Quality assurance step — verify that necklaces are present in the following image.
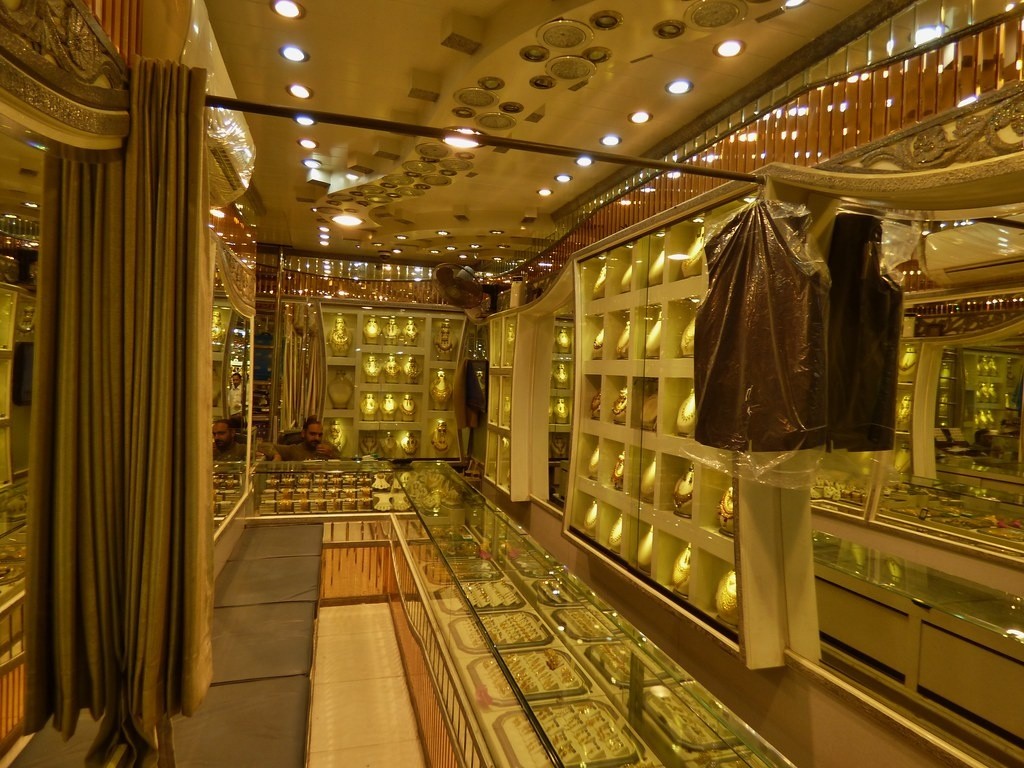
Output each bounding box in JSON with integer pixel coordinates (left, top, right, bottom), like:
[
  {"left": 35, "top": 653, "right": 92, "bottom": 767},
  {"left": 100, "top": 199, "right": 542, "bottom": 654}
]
[{"left": 331, "top": 237, "right": 737, "bottom": 612}]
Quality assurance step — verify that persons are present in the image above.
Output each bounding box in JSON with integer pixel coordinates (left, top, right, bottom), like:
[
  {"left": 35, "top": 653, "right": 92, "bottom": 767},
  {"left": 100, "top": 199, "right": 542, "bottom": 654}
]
[
  {"left": 258, "top": 415, "right": 342, "bottom": 461},
  {"left": 212, "top": 419, "right": 246, "bottom": 461},
  {"left": 228, "top": 373, "right": 248, "bottom": 421}
]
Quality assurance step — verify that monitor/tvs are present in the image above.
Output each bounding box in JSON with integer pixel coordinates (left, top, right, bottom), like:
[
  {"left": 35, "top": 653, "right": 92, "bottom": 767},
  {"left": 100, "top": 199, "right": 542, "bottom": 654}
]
[{"left": 467, "top": 359, "right": 489, "bottom": 413}]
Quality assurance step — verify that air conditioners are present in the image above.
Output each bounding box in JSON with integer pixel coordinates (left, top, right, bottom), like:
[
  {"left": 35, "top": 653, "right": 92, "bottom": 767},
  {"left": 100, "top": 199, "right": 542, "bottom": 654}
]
[{"left": 923, "top": 214, "right": 1024, "bottom": 288}]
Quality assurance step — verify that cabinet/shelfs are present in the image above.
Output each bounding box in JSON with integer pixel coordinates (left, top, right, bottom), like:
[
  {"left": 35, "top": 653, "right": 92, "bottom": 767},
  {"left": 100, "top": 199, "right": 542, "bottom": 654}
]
[
  {"left": 936, "top": 349, "right": 1024, "bottom": 432},
  {"left": 315, "top": 302, "right": 469, "bottom": 463},
  {"left": 490, "top": 173, "right": 785, "bottom": 671}
]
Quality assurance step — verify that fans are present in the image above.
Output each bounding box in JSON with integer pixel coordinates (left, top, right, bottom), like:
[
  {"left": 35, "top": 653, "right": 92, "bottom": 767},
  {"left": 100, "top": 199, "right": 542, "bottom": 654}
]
[{"left": 432, "top": 262, "right": 489, "bottom": 319}]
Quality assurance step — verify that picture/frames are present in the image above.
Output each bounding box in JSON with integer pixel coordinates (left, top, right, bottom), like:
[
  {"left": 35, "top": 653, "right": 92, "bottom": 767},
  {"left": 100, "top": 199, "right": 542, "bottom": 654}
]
[{"left": 466, "top": 360, "right": 488, "bottom": 412}]
